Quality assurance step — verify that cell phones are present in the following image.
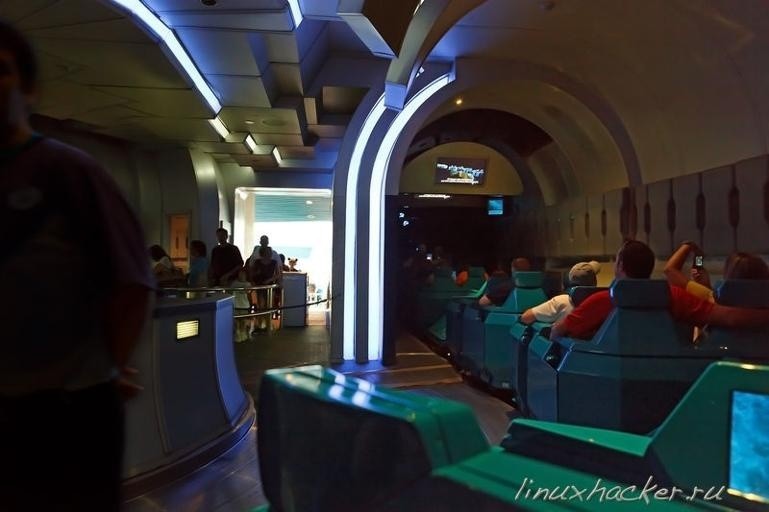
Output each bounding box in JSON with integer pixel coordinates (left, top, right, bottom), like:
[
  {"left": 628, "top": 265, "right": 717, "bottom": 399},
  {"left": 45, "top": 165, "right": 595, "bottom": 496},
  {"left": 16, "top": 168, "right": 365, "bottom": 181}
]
[{"left": 693, "top": 254, "right": 704, "bottom": 269}]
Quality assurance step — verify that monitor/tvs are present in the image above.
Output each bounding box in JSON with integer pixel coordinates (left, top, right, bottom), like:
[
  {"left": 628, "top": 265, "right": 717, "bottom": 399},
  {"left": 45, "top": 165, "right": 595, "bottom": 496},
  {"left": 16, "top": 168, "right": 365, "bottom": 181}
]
[
  {"left": 486, "top": 196, "right": 505, "bottom": 216},
  {"left": 724, "top": 387, "right": 769, "bottom": 504},
  {"left": 434, "top": 157, "right": 486, "bottom": 185}
]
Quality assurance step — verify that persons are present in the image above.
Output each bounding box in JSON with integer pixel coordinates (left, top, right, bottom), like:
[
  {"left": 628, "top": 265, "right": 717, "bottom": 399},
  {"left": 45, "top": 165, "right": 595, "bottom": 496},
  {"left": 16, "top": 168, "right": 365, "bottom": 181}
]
[
  {"left": 0, "top": 15, "right": 156, "bottom": 512},
  {"left": 149, "top": 224, "right": 292, "bottom": 343}
]
